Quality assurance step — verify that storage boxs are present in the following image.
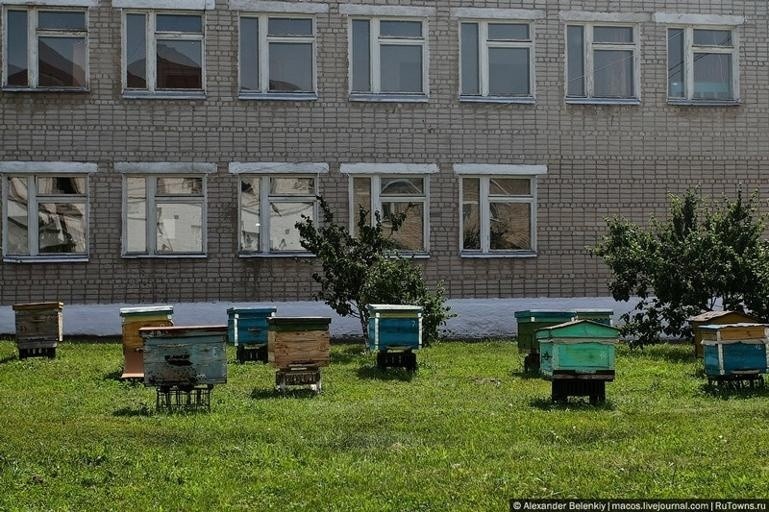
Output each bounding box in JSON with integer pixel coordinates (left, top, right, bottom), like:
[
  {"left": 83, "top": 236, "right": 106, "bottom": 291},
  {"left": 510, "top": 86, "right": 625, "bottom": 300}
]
[
  {"left": 119, "top": 304, "right": 175, "bottom": 345},
  {"left": 365, "top": 303, "right": 425, "bottom": 347},
  {"left": 227, "top": 305, "right": 277, "bottom": 344},
  {"left": 265, "top": 315, "right": 333, "bottom": 361},
  {"left": 138, "top": 323, "right": 230, "bottom": 385},
  {"left": 12, "top": 302, "right": 65, "bottom": 342},
  {"left": 516, "top": 309, "right": 614, "bottom": 352},
  {"left": 698, "top": 323, "right": 768, "bottom": 376},
  {"left": 538, "top": 319, "right": 621, "bottom": 380},
  {"left": 690, "top": 310, "right": 761, "bottom": 359}
]
